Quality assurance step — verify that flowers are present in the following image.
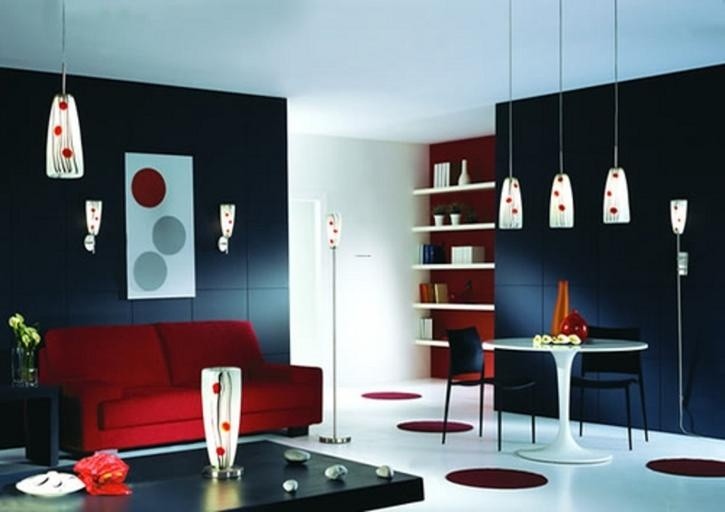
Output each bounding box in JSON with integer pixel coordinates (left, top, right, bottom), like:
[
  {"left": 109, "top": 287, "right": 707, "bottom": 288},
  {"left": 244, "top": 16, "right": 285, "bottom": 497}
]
[{"left": 8, "top": 313, "right": 42, "bottom": 379}]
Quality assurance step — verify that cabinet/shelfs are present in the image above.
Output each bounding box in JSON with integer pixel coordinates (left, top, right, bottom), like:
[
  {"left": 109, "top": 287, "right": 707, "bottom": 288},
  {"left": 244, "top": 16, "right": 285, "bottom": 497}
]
[{"left": 412, "top": 181, "right": 495, "bottom": 352}]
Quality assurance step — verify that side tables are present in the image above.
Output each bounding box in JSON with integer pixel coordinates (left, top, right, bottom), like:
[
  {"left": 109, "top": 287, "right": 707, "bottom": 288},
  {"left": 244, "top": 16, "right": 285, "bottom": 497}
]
[{"left": 0, "top": 383, "right": 60, "bottom": 467}]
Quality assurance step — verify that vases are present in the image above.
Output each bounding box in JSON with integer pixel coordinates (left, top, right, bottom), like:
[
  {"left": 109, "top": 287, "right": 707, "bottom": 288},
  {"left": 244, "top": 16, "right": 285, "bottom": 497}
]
[
  {"left": 458, "top": 160, "right": 471, "bottom": 185},
  {"left": 11, "top": 347, "right": 39, "bottom": 389},
  {"left": 562, "top": 308, "right": 589, "bottom": 343},
  {"left": 551, "top": 281, "right": 569, "bottom": 337}
]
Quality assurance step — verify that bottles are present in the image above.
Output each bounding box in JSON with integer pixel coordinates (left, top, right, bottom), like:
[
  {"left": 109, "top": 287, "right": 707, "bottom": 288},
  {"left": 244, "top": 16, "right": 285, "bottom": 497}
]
[
  {"left": 459, "top": 160, "right": 470, "bottom": 186},
  {"left": 554, "top": 281, "right": 569, "bottom": 335}
]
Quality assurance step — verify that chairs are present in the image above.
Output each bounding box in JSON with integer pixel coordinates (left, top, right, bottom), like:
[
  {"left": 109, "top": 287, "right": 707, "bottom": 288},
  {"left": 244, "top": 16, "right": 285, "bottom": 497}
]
[
  {"left": 572, "top": 327, "right": 648, "bottom": 450},
  {"left": 443, "top": 326, "right": 537, "bottom": 451}
]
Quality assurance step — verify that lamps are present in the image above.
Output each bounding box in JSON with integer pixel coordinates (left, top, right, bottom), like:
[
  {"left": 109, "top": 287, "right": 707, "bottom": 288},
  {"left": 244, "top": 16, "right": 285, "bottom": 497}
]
[
  {"left": 498, "top": 0, "right": 523, "bottom": 229},
  {"left": 217, "top": 204, "right": 236, "bottom": 252},
  {"left": 199, "top": 368, "right": 245, "bottom": 479},
  {"left": 83, "top": 200, "right": 103, "bottom": 255},
  {"left": 320, "top": 212, "right": 351, "bottom": 443},
  {"left": 671, "top": 199, "right": 688, "bottom": 433},
  {"left": 45, "top": 0, "right": 85, "bottom": 179},
  {"left": 548, "top": 0, "right": 574, "bottom": 229},
  {"left": 602, "top": 0, "right": 632, "bottom": 227}
]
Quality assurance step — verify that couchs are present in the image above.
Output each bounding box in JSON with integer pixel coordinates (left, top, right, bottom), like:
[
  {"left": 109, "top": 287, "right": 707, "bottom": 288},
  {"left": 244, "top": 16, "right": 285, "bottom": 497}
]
[{"left": 39, "top": 319, "right": 322, "bottom": 460}]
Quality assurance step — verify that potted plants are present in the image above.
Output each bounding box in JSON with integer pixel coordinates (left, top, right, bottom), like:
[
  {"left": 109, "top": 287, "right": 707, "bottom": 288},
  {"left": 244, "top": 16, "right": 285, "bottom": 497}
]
[
  {"left": 446, "top": 201, "right": 463, "bottom": 225},
  {"left": 432, "top": 206, "right": 445, "bottom": 226}
]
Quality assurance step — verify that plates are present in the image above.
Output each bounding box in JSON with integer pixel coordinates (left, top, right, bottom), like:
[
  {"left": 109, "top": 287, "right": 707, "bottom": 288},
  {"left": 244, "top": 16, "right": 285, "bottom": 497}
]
[{"left": 15, "top": 472, "right": 84, "bottom": 499}]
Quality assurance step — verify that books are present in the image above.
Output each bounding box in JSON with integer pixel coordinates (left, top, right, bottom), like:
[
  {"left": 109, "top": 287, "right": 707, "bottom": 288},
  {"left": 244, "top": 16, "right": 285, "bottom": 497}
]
[
  {"left": 451, "top": 245, "right": 486, "bottom": 265},
  {"left": 420, "top": 283, "right": 449, "bottom": 303},
  {"left": 419, "top": 244, "right": 446, "bottom": 264},
  {"left": 420, "top": 318, "right": 442, "bottom": 340},
  {"left": 433, "top": 162, "right": 460, "bottom": 189}
]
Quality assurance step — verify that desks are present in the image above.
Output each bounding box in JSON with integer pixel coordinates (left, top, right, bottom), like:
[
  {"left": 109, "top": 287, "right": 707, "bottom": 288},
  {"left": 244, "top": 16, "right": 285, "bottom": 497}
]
[
  {"left": 0, "top": 439, "right": 424, "bottom": 512},
  {"left": 481, "top": 338, "right": 649, "bottom": 464}
]
